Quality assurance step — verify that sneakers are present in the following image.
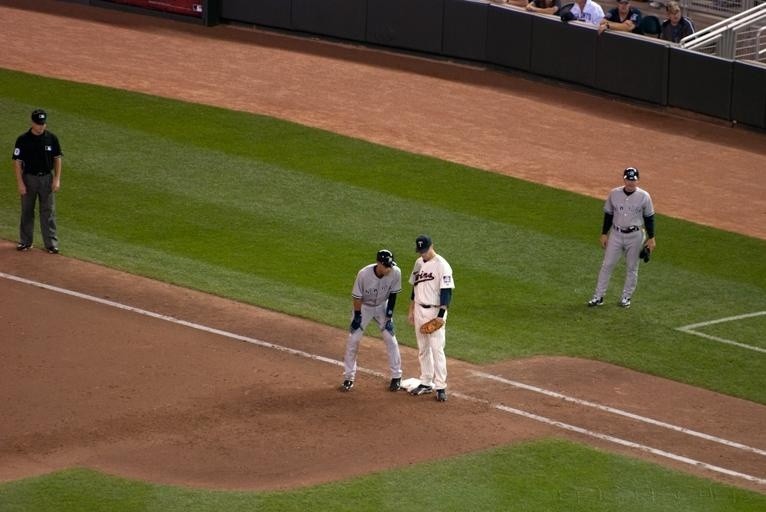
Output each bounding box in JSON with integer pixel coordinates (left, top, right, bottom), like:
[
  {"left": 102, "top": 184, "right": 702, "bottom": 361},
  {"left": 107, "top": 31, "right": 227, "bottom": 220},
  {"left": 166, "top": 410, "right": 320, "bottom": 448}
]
[
  {"left": 390, "top": 378, "right": 401, "bottom": 392},
  {"left": 617, "top": 297, "right": 631, "bottom": 306},
  {"left": 45, "top": 244, "right": 59, "bottom": 254},
  {"left": 17, "top": 242, "right": 34, "bottom": 251},
  {"left": 409, "top": 384, "right": 433, "bottom": 396},
  {"left": 342, "top": 379, "right": 354, "bottom": 391},
  {"left": 587, "top": 296, "right": 605, "bottom": 306},
  {"left": 435, "top": 387, "right": 448, "bottom": 402}
]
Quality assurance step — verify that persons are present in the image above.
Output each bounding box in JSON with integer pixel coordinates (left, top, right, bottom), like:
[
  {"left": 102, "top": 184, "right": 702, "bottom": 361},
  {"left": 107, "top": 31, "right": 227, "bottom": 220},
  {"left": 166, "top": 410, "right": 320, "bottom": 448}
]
[
  {"left": 339, "top": 249, "right": 404, "bottom": 393},
  {"left": 588, "top": 166, "right": 656, "bottom": 310},
  {"left": 526, "top": 0, "right": 561, "bottom": 16},
  {"left": 597, "top": 0, "right": 644, "bottom": 38},
  {"left": 407, "top": 234, "right": 456, "bottom": 402},
  {"left": 661, "top": 0, "right": 697, "bottom": 44},
  {"left": 12, "top": 109, "right": 64, "bottom": 254},
  {"left": 570, "top": 0, "right": 605, "bottom": 26}
]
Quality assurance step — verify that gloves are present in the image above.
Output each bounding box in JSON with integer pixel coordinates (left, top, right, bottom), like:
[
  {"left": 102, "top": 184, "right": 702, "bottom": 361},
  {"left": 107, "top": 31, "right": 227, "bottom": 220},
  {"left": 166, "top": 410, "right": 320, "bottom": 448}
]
[
  {"left": 349, "top": 310, "right": 363, "bottom": 334},
  {"left": 384, "top": 317, "right": 396, "bottom": 336}
]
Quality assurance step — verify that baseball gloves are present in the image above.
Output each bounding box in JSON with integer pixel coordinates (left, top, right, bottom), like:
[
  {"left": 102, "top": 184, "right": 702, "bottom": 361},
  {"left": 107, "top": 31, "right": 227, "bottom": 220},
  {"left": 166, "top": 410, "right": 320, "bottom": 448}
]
[
  {"left": 420, "top": 318, "right": 443, "bottom": 334},
  {"left": 638, "top": 243, "right": 653, "bottom": 262}
]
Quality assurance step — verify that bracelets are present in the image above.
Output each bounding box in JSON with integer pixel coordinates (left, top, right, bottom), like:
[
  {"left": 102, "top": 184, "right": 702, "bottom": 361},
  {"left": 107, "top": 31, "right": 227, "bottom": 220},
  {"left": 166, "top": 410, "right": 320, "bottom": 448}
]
[{"left": 437, "top": 308, "right": 445, "bottom": 318}]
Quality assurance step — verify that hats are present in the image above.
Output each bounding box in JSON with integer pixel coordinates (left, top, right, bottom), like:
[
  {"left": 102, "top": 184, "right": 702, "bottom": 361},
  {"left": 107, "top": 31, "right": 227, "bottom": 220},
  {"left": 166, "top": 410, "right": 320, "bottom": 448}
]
[
  {"left": 623, "top": 166, "right": 639, "bottom": 181},
  {"left": 31, "top": 109, "right": 46, "bottom": 125},
  {"left": 376, "top": 250, "right": 396, "bottom": 268},
  {"left": 561, "top": 10, "right": 578, "bottom": 22},
  {"left": 416, "top": 233, "right": 432, "bottom": 253}
]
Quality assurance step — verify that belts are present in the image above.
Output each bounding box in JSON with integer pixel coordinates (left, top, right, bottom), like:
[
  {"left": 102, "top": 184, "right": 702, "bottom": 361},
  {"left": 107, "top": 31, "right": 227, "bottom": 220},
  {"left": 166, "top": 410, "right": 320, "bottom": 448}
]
[{"left": 613, "top": 225, "right": 642, "bottom": 232}]
